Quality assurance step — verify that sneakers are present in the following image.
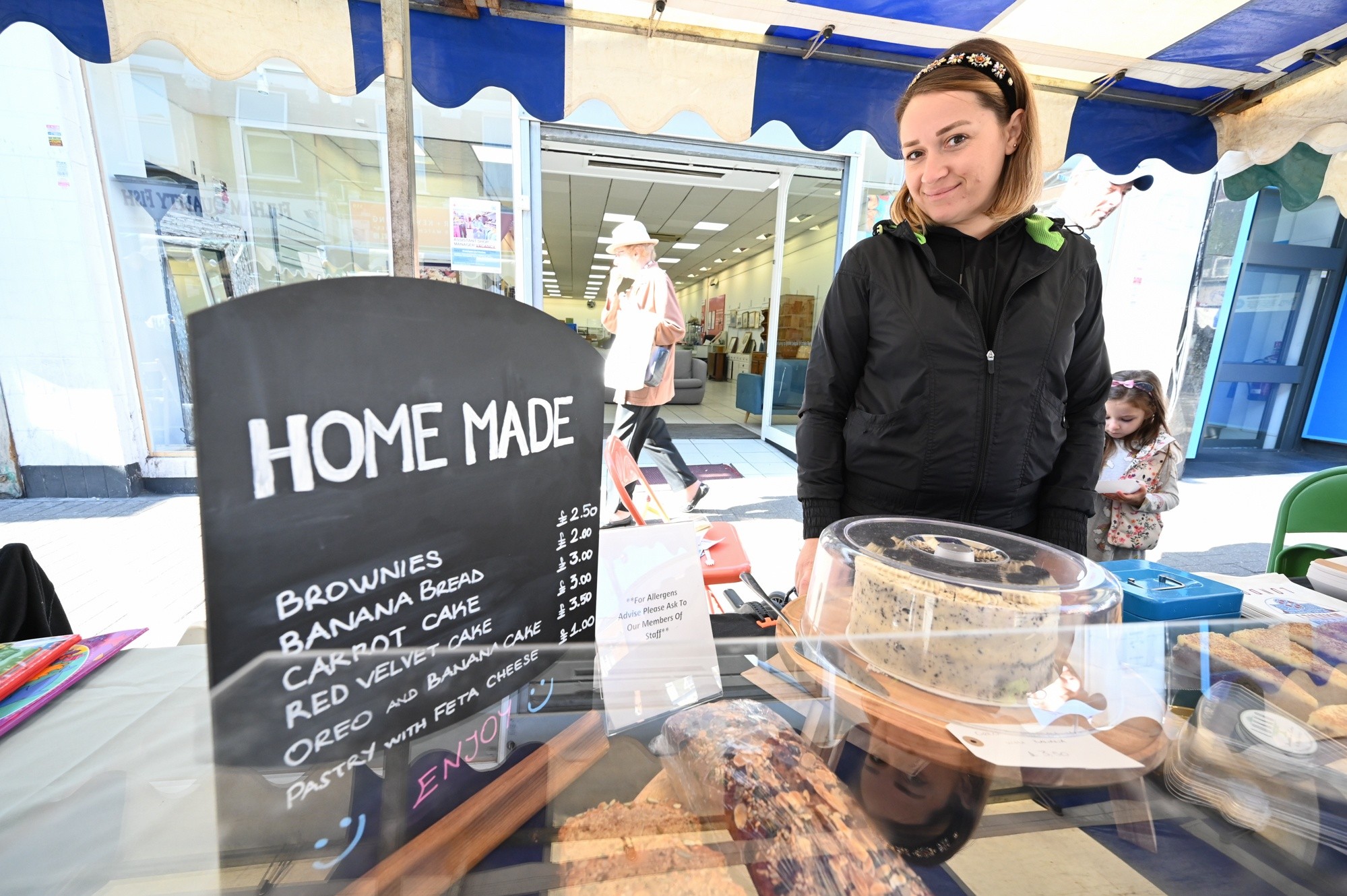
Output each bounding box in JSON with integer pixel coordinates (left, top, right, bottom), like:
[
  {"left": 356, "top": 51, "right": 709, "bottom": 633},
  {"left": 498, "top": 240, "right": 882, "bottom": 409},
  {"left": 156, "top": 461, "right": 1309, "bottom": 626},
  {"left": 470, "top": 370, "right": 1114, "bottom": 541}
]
[
  {"left": 598, "top": 513, "right": 634, "bottom": 529},
  {"left": 681, "top": 482, "right": 709, "bottom": 513}
]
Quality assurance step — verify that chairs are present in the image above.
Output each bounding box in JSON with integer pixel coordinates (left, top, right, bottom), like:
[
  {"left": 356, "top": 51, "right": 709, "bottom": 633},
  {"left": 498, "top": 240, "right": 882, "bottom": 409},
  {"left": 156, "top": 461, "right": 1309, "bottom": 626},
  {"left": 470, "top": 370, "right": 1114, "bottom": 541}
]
[
  {"left": 605, "top": 434, "right": 750, "bottom": 615},
  {"left": 1264, "top": 467, "right": 1347, "bottom": 578}
]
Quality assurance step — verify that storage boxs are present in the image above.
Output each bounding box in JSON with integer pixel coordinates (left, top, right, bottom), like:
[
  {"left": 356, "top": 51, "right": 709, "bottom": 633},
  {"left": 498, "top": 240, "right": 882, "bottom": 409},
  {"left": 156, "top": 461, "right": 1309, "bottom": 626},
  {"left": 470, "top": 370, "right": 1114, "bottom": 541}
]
[
  {"left": 1098, "top": 560, "right": 1245, "bottom": 627},
  {"left": 583, "top": 334, "right": 599, "bottom": 347}
]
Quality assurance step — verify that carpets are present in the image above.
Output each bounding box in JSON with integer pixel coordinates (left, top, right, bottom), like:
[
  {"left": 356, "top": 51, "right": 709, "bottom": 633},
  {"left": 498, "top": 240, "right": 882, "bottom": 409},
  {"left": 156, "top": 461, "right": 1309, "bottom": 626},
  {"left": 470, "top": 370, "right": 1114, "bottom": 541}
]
[
  {"left": 633, "top": 462, "right": 745, "bottom": 485},
  {"left": 603, "top": 424, "right": 761, "bottom": 439}
]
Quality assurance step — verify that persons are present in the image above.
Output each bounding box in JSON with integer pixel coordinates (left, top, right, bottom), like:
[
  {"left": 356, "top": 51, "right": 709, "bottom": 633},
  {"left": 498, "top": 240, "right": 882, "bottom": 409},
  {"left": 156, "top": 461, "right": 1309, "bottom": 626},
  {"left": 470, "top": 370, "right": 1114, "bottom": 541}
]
[
  {"left": 835, "top": 711, "right": 985, "bottom": 868},
  {"left": 794, "top": 39, "right": 1113, "bottom": 600},
  {"left": 1087, "top": 370, "right": 1184, "bottom": 562},
  {"left": 600, "top": 222, "right": 710, "bottom": 530},
  {"left": 1040, "top": 156, "right": 1155, "bottom": 240},
  {"left": 501, "top": 220, "right": 515, "bottom": 298},
  {"left": 454, "top": 211, "right": 486, "bottom": 239}
]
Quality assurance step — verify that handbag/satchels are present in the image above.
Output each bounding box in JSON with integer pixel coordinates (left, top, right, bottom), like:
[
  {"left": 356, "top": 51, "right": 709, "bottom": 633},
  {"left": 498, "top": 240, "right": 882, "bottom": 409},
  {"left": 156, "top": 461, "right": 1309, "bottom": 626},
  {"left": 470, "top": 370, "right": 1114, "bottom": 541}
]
[{"left": 645, "top": 343, "right": 675, "bottom": 388}]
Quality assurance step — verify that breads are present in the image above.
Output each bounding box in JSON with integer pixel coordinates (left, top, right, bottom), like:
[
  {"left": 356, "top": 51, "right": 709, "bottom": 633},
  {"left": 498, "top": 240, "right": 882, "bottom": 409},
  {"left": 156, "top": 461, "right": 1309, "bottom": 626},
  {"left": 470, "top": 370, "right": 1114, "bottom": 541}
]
[
  {"left": 548, "top": 695, "right": 931, "bottom": 896},
  {"left": 1172, "top": 619, "right": 1347, "bottom": 740}
]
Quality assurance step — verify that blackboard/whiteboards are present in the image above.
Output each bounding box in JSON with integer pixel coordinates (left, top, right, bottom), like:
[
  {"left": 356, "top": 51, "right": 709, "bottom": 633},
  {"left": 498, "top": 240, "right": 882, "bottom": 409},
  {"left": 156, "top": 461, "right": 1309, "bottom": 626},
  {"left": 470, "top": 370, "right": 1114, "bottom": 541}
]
[{"left": 186, "top": 275, "right": 603, "bottom": 896}]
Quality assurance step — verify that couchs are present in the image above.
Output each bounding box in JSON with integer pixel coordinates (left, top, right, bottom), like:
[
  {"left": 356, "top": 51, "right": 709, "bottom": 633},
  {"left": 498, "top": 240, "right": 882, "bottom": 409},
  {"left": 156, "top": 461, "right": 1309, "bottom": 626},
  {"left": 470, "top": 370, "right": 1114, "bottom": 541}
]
[
  {"left": 736, "top": 359, "right": 811, "bottom": 423},
  {"left": 666, "top": 349, "right": 707, "bottom": 405}
]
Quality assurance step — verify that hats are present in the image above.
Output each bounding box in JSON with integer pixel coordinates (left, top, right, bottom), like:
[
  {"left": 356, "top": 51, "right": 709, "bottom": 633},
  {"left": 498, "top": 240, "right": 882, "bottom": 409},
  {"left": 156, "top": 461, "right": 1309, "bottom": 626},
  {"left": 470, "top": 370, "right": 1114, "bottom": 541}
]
[{"left": 606, "top": 220, "right": 659, "bottom": 254}]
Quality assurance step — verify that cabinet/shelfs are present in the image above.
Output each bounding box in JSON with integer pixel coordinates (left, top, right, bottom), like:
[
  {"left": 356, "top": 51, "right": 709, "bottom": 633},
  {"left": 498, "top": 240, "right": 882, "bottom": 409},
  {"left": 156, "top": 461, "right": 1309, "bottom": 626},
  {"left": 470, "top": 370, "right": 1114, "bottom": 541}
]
[
  {"left": 739, "top": 362, "right": 749, "bottom": 372},
  {"left": 589, "top": 327, "right": 605, "bottom": 341},
  {"left": 727, "top": 361, "right": 732, "bottom": 378},
  {"left": 751, "top": 295, "right": 814, "bottom": 374},
  {"left": 707, "top": 352, "right": 727, "bottom": 382},
  {"left": 732, "top": 361, "right": 739, "bottom": 378}
]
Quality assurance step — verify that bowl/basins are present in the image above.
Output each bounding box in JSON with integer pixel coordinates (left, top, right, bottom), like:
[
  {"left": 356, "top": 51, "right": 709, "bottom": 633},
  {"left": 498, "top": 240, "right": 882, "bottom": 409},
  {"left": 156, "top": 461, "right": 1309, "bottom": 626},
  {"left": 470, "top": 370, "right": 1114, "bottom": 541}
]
[{"left": 1095, "top": 480, "right": 1145, "bottom": 494}]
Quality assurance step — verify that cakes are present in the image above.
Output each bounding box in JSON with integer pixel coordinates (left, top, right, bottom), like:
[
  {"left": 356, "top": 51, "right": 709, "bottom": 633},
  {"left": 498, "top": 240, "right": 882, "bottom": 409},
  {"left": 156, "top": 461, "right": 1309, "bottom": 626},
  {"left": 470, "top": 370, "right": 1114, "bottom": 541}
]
[{"left": 846, "top": 534, "right": 1060, "bottom": 705}]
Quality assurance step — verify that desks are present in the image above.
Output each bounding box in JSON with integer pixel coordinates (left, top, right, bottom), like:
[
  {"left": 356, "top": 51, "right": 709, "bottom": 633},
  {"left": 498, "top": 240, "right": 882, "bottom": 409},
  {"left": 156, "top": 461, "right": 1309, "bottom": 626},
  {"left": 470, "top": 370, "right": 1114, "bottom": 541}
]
[{"left": 0, "top": 636, "right": 331, "bottom": 896}]
